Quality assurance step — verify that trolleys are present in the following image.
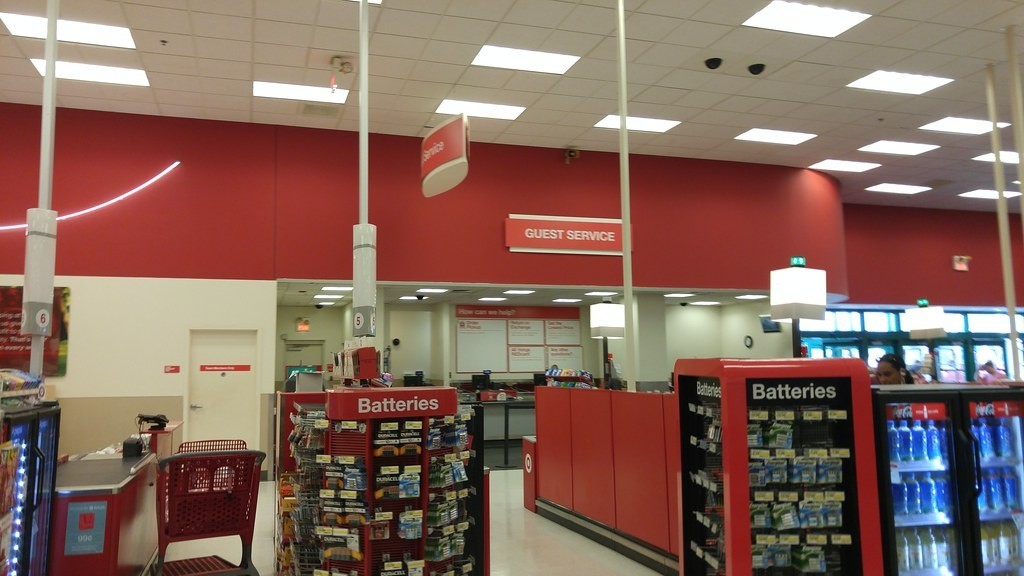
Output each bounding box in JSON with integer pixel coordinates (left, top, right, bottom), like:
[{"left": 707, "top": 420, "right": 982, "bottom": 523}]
[{"left": 150, "top": 439, "right": 267, "bottom": 576}]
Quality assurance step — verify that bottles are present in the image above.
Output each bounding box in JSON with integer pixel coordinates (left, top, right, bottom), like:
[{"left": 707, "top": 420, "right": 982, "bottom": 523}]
[
  {"left": 896, "top": 528, "right": 951, "bottom": 570},
  {"left": 888, "top": 419, "right": 948, "bottom": 461},
  {"left": 981, "top": 521, "right": 1019, "bottom": 566},
  {"left": 891, "top": 471, "right": 952, "bottom": 515},
  {"left": 971, "top": 417, "right": 1014, "bottom": 461},
  {"left": 975, "top": 467, "right": 1018, "bottom": 511}
]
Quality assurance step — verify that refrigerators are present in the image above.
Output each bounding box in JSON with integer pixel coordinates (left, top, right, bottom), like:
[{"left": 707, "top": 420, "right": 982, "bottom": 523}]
[{"left": 871, "top": 384, "right": 1024, "bottom": 576}]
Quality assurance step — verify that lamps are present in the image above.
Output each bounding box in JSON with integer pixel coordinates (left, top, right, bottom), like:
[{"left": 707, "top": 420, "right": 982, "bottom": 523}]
[
  {"left": 905, "top": 306, "right": 946, "bottom": 381},
  {"left": 769, "top": 266, "right": 827, "bottom": 358},
  {"left": 590, "top": 303, "right": 625, "bottom": 388}
]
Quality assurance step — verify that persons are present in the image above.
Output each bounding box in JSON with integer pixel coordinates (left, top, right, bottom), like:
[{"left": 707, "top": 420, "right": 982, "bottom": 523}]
[
  {"left": 877, "top": 352, "right": 915, "bottom": 384},
  {"left": 978, "top": 360, "right": 1008, "bottom": 383}
]
